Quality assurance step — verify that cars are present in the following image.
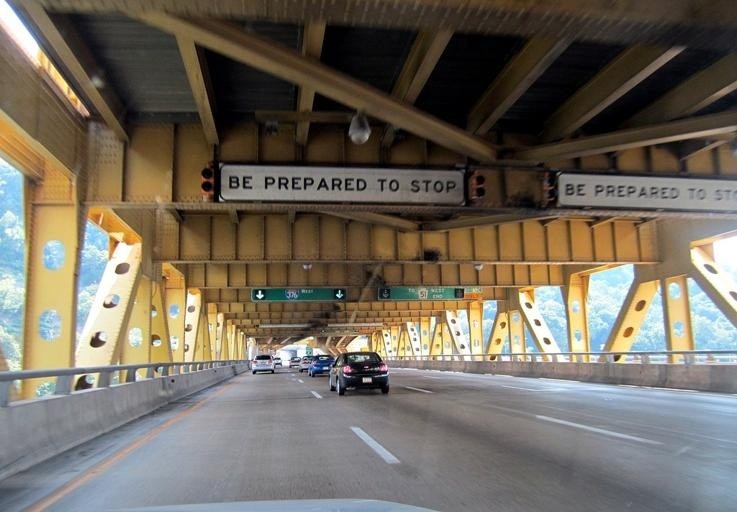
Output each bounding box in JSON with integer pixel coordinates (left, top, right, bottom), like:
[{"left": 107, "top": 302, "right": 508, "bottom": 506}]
[
  {"left": 251, "top": 354, "right": 335, "bottom": 377},
  {"left": 327, "top": 352, "right": 389, "bottom": 397}
]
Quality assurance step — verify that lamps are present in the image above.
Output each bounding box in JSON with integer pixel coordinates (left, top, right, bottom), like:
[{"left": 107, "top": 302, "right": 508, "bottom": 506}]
[{"left": 347, "top": 110, "right": 372, "bottom": 146}]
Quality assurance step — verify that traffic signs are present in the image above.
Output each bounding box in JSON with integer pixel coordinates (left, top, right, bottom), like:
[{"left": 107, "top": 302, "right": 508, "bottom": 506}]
[
  {"left": 376, "top": 286, "right": 483, "bottom": 301},
  {"left": 250, "top": 287, "right": 351, "bottom": 301}
]
